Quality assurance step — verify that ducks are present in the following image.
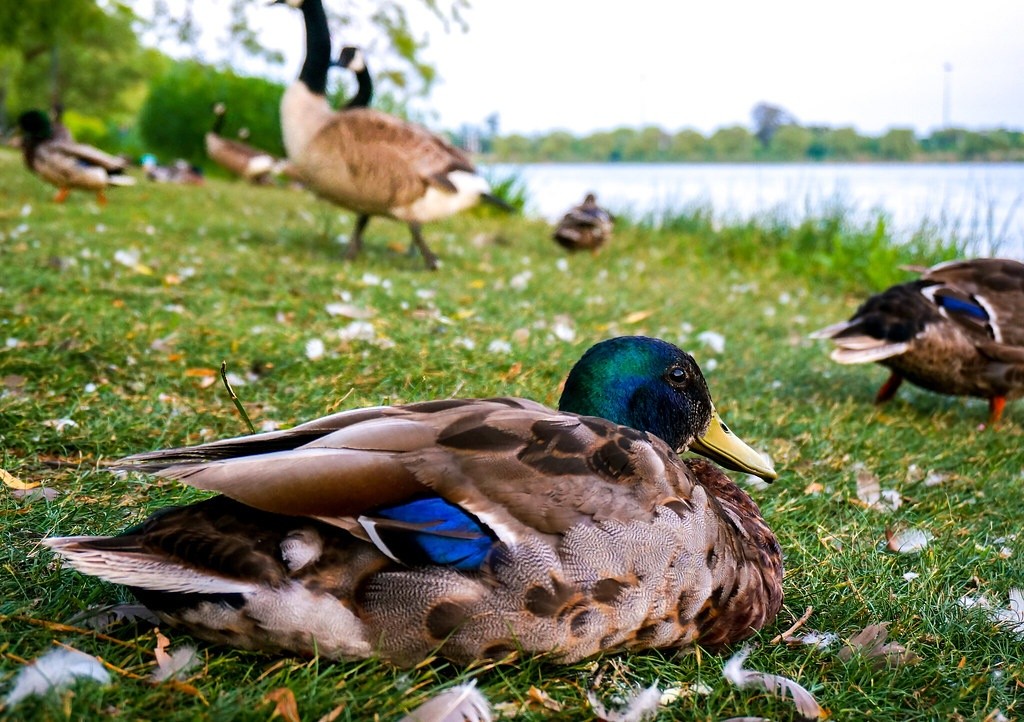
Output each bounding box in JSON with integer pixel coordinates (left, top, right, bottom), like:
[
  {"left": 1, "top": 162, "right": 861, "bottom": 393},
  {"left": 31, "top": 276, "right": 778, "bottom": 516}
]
[
  {"left": 805, "top": 258, "right": 1023, "bottom": 433},
  {"left": 33, "top": 331, "right": 785, "bottom": 668}
]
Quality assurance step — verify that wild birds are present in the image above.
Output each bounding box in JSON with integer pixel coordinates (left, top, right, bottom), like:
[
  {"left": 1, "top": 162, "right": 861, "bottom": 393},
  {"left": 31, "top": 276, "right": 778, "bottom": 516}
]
[
  {"left": 1, "top": 107, "right": 139, "bottom": 209},
  {"left": 263, "top": 0, "right": 520, "bottom": 273},
  {"left": 204, "top": 99, "right": 287, "bottom": 192},
  {"left": 549, "top": 193, "right": 617, "bottom": 255}
]
